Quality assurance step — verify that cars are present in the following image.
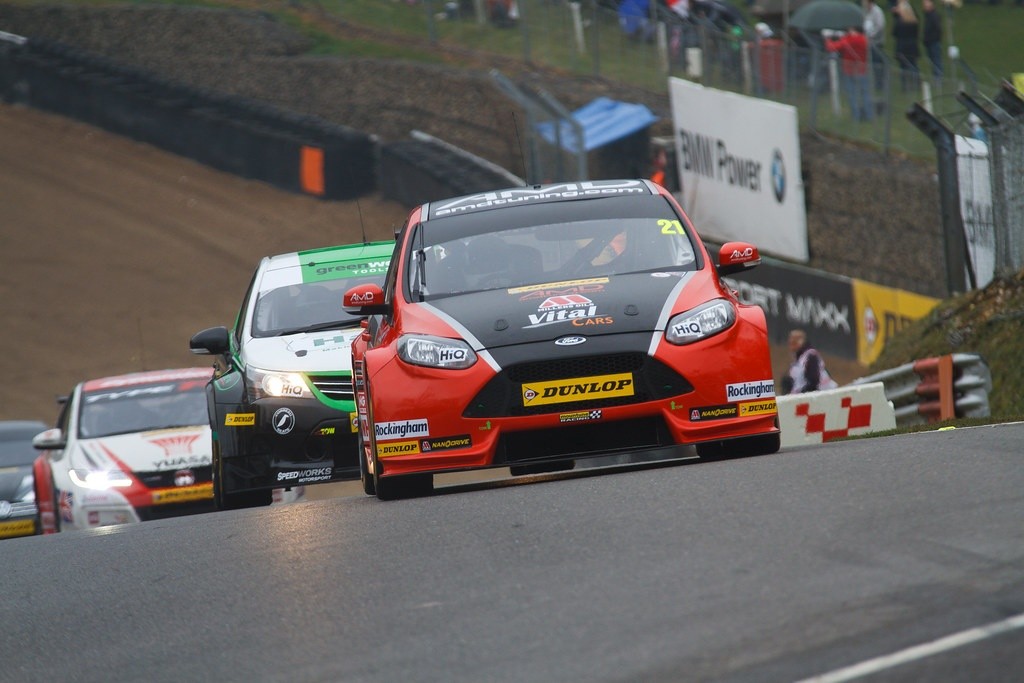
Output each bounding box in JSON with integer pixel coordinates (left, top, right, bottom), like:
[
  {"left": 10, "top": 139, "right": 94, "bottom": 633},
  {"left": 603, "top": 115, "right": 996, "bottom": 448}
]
[
  {"left": 187, "top": 239, "right": 400, "bottom": 510},
  {"left": 31, "top": 365, "right": 306, "bottom": 534},
  {"left": 0, "top": 420, "right": 55, "bottom": 540},
  {"left": 340, "top": 177, "right": 781, "bottom": 500}
]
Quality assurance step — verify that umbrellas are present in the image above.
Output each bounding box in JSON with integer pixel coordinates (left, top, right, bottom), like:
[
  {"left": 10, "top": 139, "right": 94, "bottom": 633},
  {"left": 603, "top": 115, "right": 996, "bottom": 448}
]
[{"left": 789, "top": 0, "right": 863, "bottom": 30}]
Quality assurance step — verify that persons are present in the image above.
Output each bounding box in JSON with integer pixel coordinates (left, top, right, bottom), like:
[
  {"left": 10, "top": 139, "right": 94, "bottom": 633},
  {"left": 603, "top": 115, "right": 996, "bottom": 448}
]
[
  {"left": 788, "top": 329, "right": 839, "bottom": 394},
  {"left": 730, "top": 22, "right": 784, "bottom": 101},
  {"left": 891, "top": 0, "right": 920, "bottom": 93},
  {"left": 860, "top": 0, "right": 887, "bottom": 92},
  {"left": 921, "top": 0, "right": 944, "bottom": 76},
  {"left": 825, "top": 26, "right": 875, "bottom": 120}
]
